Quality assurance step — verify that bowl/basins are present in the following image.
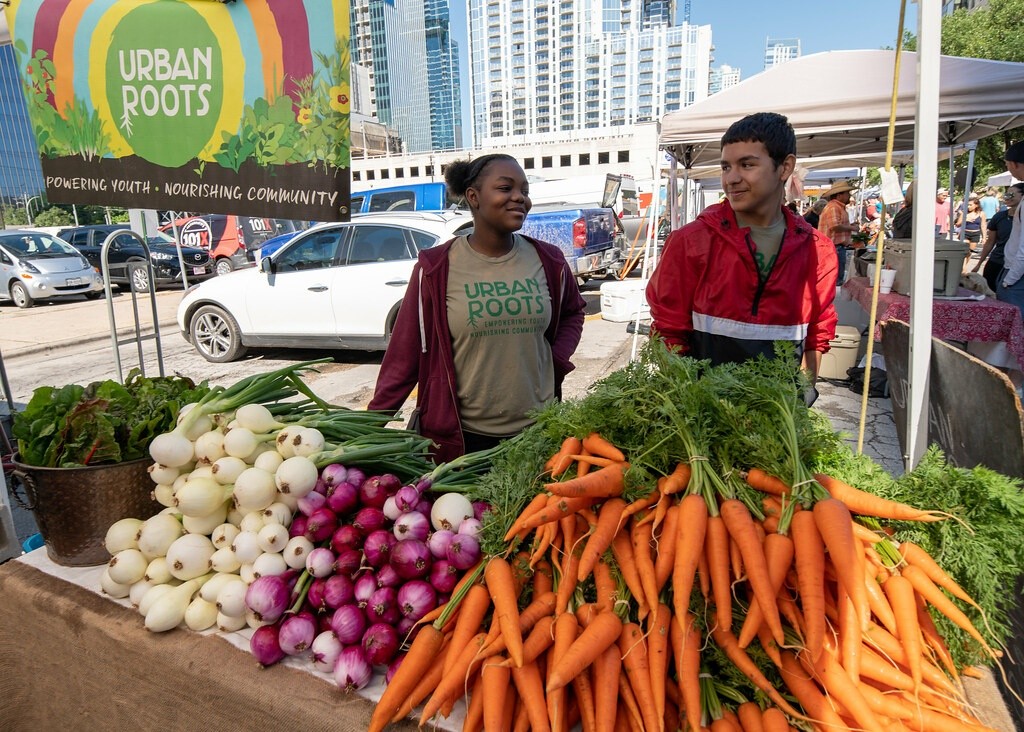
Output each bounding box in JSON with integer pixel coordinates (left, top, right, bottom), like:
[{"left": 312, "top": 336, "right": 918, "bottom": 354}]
[{"left": 935, "top": 225, "right": 948, "bottom": 240}]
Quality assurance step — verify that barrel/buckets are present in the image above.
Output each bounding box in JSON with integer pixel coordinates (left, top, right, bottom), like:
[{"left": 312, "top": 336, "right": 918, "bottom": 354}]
[{"left": 8, "top": 450, "right": 161, "bottom": 570}]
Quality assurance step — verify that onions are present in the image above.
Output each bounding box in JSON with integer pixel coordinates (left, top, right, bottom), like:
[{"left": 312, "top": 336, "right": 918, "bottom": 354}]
[{"left": 101, "top": 402, "right": 490, "bottom": 691}]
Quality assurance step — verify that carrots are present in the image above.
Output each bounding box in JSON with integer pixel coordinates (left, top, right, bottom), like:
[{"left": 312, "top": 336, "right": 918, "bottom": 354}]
[{"left": 367, "top": 435, "right": 1024, "bottom": 732}]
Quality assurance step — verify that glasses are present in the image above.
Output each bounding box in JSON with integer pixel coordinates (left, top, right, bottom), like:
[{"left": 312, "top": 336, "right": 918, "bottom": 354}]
[{"left": 1003, "top": 192, "right": 1015, "bottom": 200}]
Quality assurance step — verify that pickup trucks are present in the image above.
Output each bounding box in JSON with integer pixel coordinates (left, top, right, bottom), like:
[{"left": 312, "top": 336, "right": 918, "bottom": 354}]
[
  {"left": 615, "top": 216, "right": 672, "bottom": 272},
  {"left": 255, "top": 182, "right": 626, "bottom": 296}
]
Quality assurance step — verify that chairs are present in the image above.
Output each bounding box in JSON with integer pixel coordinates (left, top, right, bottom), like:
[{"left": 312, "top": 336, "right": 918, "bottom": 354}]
[
  {"left": 379, "top": 238, "right": 406, "bottom": 259},
  {"left": 353, "top": 242, "right": 374, "bottom": 262}
]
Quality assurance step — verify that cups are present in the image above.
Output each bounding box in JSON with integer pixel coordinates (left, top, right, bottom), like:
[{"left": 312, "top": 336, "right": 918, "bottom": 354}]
[
  {"left": 869, "top": 264, "right": 885, "bottom": 287},
  {"left": 879, "top": 269, "right": 898, "bottom": 294}
]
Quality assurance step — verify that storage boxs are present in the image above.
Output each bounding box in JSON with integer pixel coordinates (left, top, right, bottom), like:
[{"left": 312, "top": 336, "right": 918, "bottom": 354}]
[
  {"left": 884, "top": 238, "right": 970, "bottom": 296},
  {"left": 600, "top": 279, "right": 651, "bottom": 322},
  {"left": 819, "top": 326, "right": 861, "bottom": 379}
]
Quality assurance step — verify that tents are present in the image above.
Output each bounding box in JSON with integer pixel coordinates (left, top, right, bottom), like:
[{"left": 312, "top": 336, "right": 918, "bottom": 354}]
[
  {"left": 629, "top": 139, "right": 979, "bottom": 385},
  {"left": 653, "top": 49, "right": 1024, "bottom": 378},
  {"left": 988, "top": 171, "right": 1023, "bottom": 187}
]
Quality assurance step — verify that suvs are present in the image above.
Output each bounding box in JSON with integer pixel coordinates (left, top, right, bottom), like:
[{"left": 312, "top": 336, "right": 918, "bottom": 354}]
[{"left": 176, "top": 211, "right": 479, "bottom": 363}]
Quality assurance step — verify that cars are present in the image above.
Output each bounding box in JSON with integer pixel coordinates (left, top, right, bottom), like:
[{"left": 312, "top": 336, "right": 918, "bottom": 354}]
[
  {"left": 48, "top": 224, "right": 216, "bottom": 294},
  {"left": 0, "top": 230, "right": 104, "bottom": 309},
  {"left": 152, "top": 213, "right": 306, "bottom": 279}
]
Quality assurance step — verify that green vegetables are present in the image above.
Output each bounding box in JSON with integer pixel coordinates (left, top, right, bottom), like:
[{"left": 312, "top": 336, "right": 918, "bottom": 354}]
[{"left": 12, "top": 367, "right": 210, "bottom": 468}]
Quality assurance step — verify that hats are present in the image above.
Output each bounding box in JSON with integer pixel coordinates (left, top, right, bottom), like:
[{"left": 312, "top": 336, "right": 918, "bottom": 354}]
[
  {"left": 1005, "top": 140, "right": 1024, "bottom": 164},
  {"left": 820, "top": 180, "right": 854, "bottom": 199},
  {"left": 937, "top": 187, "right": 949, "bottom": 195}
]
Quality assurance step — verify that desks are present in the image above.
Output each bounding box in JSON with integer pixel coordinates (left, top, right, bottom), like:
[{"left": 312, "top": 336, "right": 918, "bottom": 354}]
[
  {"left": 853, "top": 257, "right": 875, "bottom": 276},
  {"left": 841, "top": 276, "right": 1024, "bottom": 398}
]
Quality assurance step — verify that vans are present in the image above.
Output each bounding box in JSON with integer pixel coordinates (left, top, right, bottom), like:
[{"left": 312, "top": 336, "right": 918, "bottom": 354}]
[{"left": 526, "top": 173, "right": 641, "bottom": 219}]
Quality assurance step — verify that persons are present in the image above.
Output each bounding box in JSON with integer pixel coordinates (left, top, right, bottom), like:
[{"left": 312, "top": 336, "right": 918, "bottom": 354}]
[
  {"left": 893, "top": 182, "right": 912, "bottom": 238},
  {"left": 935, "top": 187, "right": 950, "bottom": 239},
  {"left": 971, "top": 183, "right": 1024, "bottom": 297},
  {"left": 956, "top": 187, "right": 1012, "bottom": 274},
  {"left": 818, "top": 181, "right": 860, "bottom": 286},
  {"left": 366, "top": 154, "right": 588, "bottom": 473},
  {"left": 1003, "top": 140, "right": 1024, "bottom": 324},
  {"left": 645, "top": 113, "right": 838, "bottom": 408},
  {"left": 806, "top": 203, "right": 810, "bottom": 212},
  {"left": 846, "top": 196, "right": 855, "bottom": 224},
  {"left": 867, "top": 200, "right": 877, "bottom": 221},
  {"left": 787, "top": 200, "right": 797, "bottom": 212},
  {"left": 876, "top": 197, "right": 883, "bottom": 212},
  {"left": 803, "top": 199, "right": 828, "bottom": 229}
]
